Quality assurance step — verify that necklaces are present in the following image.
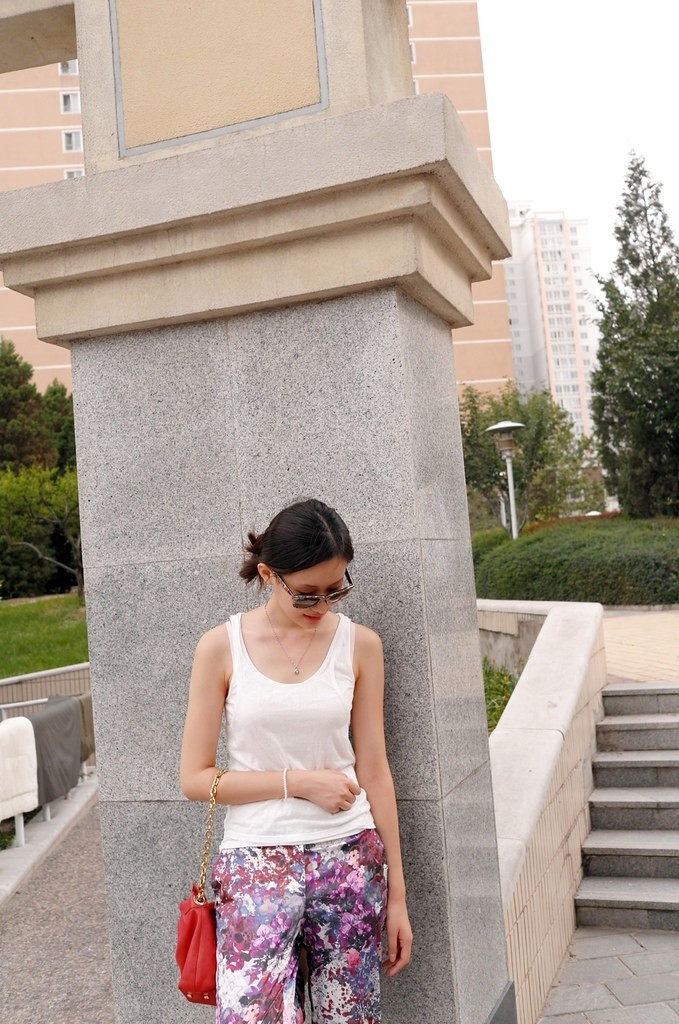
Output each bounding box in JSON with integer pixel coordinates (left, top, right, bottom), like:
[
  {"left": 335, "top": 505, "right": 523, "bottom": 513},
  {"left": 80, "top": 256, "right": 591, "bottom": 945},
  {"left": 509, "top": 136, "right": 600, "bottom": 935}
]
[{"left": 265, "top": 598, "right": 316, "bottom": 676}]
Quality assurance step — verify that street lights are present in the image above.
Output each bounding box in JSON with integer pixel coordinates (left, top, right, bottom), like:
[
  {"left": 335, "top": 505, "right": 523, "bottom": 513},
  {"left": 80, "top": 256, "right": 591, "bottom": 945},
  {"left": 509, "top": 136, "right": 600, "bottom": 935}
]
[{"left": 482, "top": 420, "right": 527, "bottom": 538}]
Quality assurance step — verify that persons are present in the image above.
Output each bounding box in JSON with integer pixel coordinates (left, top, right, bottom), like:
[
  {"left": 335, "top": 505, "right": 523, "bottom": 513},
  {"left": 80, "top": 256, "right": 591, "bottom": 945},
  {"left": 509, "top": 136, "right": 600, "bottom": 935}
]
[{"left": 180, "top": 499, "right": 414, "bottom": 1024}]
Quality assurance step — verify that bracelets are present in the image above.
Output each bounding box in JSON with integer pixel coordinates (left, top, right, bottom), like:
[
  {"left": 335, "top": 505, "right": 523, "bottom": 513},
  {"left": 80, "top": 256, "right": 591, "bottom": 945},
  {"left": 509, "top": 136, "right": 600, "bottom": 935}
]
[{"left": 283, "top": 767, "right": 288, "bottom": 800}]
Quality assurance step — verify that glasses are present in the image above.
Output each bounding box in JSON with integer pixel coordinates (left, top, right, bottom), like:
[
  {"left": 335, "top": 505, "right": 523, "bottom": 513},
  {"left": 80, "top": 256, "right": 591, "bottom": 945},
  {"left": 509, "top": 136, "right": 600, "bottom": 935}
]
[{"left": 270, "top": 568, "right": 355, "bottom": 609}]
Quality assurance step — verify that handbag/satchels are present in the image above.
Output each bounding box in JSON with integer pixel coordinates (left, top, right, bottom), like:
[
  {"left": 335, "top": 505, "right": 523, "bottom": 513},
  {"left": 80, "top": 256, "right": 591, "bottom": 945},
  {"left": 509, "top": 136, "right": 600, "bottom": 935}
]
[{"left": 176, "top": 882, "right": 216, "bottom": 1005}]
